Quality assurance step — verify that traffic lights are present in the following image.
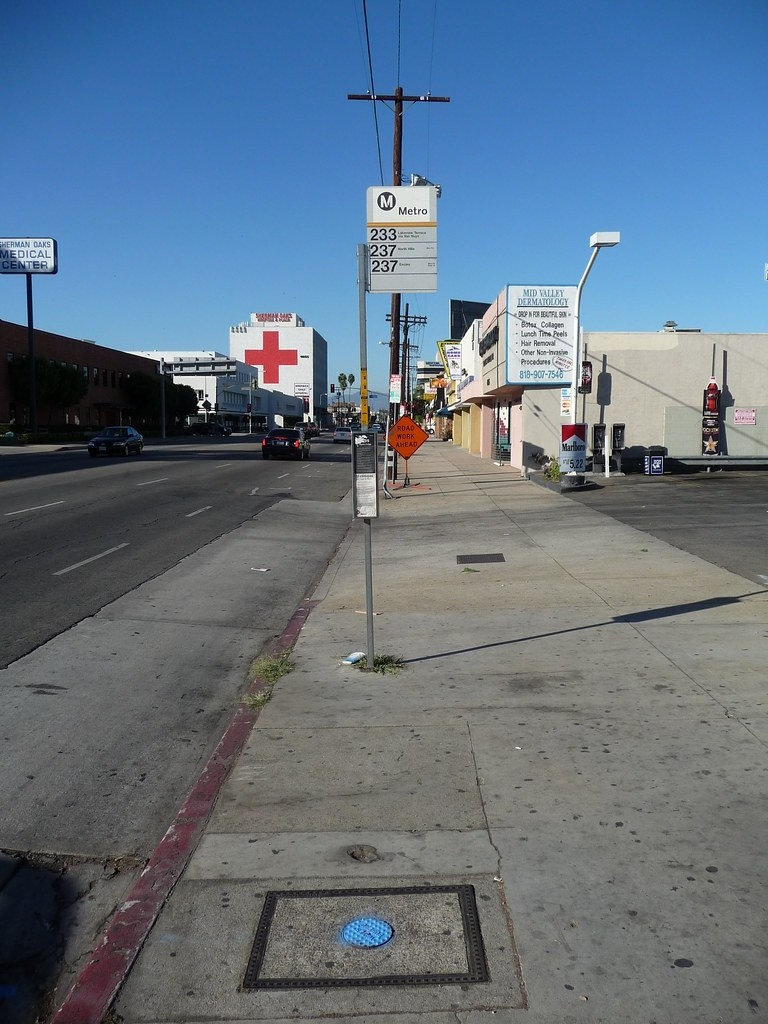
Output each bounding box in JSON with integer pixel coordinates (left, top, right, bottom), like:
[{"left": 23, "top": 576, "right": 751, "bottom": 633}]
[
  {"left": 330, "top": 384, "right": 334, "bottom": 395},
  {"left": 253, "top": 380, "right": 261, "bottom": 391}
]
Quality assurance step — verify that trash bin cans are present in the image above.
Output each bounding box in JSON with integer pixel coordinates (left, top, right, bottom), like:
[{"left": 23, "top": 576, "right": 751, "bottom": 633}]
[{"left": 642, "top": 449, "right": 665, "bottom": 476}]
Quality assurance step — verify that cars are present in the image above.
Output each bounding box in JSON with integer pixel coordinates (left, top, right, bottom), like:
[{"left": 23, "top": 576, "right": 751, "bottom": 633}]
[
  {"left": 371, "top": 424, "right": 381, "bottom": 432},
  {"left": 308, "top": 421, "right": 320, "bottom": 436},
  {"left": 293, "top": 422, "right": 312, "bottom": 440},
  {"left": 88, "top": 426, "right": 146, "bottom": 457},
  {"left": 261, "top": 427, "right": 312, "bottom": 462},
  {"left": 333, "top": 426, "right": 352, "bottom": 443}
]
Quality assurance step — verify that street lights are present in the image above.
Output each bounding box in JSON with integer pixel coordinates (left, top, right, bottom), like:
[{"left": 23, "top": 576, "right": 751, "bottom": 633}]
[{"left": 559, "top": 232, "right": 622, "bottom": 487}]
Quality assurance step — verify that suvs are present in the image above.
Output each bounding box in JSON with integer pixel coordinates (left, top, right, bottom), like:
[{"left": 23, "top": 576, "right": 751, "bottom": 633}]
[{"left": 189, "top": 421, "right": 231, "bottom": 438}]
